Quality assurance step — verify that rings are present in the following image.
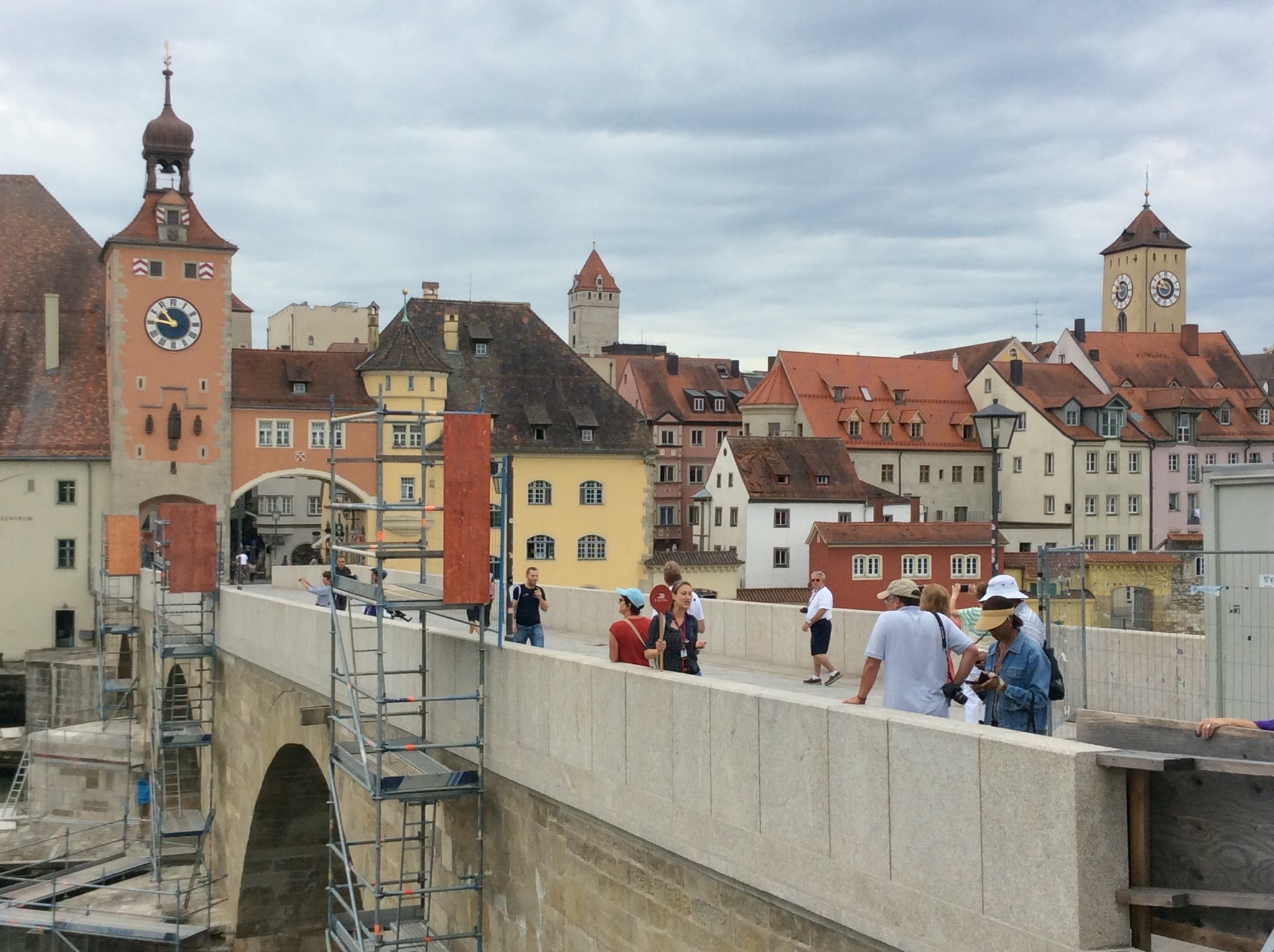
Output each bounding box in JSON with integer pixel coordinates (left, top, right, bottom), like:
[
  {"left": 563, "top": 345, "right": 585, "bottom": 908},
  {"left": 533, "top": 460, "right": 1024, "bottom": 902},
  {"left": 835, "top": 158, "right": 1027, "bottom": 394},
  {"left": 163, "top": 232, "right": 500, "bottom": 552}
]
[{"left": 1207, "top": 724, "right": 1211, "bottom": 727}]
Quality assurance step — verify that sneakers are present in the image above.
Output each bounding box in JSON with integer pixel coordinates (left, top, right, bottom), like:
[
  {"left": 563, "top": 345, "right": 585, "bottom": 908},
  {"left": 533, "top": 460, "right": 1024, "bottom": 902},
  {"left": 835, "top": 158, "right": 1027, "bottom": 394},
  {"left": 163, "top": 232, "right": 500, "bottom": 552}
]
[
  {"left": 803, "top": 678, "right": 822, "bottom": 684},
  {"left": 825, "top": 671, "right": 843, "bottom": 685}
]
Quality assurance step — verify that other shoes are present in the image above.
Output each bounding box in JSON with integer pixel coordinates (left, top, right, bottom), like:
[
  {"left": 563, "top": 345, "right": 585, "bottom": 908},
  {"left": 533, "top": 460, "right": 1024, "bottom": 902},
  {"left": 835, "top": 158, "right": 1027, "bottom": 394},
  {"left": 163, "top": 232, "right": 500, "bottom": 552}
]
[{"left": 469, "top": 621, "right": 475, "bottom": 634}]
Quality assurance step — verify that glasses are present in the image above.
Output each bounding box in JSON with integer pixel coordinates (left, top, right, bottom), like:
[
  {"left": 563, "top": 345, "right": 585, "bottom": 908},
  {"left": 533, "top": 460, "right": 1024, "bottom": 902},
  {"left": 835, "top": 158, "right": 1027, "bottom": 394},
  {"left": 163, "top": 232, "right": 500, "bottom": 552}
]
[
  {"left": 812, "top": 578, "right": 823, "bottom": 582},
  {"left": 884, "top": 597, "right": 895, "bottom": 605},
  {"left": 619, "top": 598, "right": 625, "bottom": 603}
]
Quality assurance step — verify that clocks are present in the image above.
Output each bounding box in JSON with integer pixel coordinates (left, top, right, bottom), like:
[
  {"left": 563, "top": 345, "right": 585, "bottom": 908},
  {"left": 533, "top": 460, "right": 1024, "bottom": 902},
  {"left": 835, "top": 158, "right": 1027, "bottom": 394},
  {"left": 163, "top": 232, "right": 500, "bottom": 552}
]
[
  {"left": 1111, "top": 274, "right": 1134, "bottom": 309},
  {"left": 145, "top": 296, "right": 203, "bottom": 351},
  {"left": 1149, "top": 270, "right": 1180, "bottom": 306}
]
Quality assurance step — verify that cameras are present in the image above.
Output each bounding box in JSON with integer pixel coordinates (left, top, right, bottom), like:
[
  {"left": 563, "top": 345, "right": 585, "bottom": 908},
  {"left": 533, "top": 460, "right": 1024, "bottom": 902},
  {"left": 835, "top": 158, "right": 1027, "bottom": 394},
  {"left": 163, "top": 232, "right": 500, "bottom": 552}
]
[
  {"left": 943, "top": 683, "right": 968, "bottom": 704},
  {"left": 299, "top": 579, "right": 302, "bottom": 581},
  {"left": 800, "top": 607, "right": 808, "bottom": 613}
]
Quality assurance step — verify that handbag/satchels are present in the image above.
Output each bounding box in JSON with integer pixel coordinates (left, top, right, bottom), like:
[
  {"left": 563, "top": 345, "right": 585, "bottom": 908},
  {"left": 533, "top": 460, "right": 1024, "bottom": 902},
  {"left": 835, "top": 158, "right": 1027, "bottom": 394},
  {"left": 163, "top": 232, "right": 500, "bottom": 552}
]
[{"left": 1040, "top": 640, "right": 1064, "bottom": 701}]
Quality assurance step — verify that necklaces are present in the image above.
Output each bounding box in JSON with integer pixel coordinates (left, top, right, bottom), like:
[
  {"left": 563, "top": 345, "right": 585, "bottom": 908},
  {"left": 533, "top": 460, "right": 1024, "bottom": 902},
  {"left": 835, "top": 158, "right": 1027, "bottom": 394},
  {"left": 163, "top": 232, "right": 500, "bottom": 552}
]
[{"left": 679, "top": 628, "right": 690, "bottom": 643}]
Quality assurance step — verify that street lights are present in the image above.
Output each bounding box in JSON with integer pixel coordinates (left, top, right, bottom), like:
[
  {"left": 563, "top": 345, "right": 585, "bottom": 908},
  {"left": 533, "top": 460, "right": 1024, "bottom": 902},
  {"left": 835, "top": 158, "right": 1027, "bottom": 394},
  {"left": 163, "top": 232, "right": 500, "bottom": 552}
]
[
  {"left": 269, "top": 507, "right": 281, "bottom": 566},
  {"left": 970, "top": 397, "right": 1021, "bottom": 577}
]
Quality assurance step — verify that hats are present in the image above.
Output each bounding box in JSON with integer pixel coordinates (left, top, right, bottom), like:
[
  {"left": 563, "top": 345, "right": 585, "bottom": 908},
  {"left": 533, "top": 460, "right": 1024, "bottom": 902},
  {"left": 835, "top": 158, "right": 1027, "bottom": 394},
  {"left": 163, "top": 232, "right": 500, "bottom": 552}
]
[
  {"left": 975, "top": 606, "right": 1015, "bottom": 630},
  {"left": 978, "top": 574, "right": 1030, "bottom": 602},
  {"left": 617, "top": 588, "right": 645, "bottom": 609},
  {"left": 877, "top": 579, "right": 920, "bottom": 600},
  {"left": 371, "top": 568, "right": 387, "bottom": 579}
]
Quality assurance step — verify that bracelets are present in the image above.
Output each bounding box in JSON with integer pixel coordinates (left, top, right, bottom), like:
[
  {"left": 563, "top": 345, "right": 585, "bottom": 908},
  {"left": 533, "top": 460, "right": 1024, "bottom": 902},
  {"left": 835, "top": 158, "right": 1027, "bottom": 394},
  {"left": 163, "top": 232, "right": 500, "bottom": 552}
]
[
  {"left": 952, "top": 597, "right": 957, "bottom": 600},
  {"left": 857, "top": 695, "right": 867, "bottom": 701},
  {"left": 806, "top": 620, "right": 812, "bottom": 626}
]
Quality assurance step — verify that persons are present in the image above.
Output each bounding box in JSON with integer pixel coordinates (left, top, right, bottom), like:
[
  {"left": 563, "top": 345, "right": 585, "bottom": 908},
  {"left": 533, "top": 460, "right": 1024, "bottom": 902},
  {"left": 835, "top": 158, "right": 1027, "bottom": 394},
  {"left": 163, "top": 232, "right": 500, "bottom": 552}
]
[
  {"left": 363, "top": 567, "right": 387, "bottom": 617},
  {"left": 842, "top": 579, "right": 979, "bottom": 718},
  {"left": 950, "top": 583, "right": 996, "bottom": 723},
  {"left": 466, "top": 573, "right": 495, "bottom": 634},
  {"left": 283, "top": 555, "right": 287, "bottom": 565},
  {"left": 968, "top": 596, "right": 1051, "bottom": 736},
  {"left": 609, "top": 561, "right": 707, "bottom": 675},
  {"left": 920, "top": 583, "right": 961, "bottom": 684},
  {"left": 512, "top": 567, "right": 548, "bottom": 647},
  {"left": 301, "top": 555, "right": 351, "bottom": 611},
  {"left": 802, "top": 571, "right": 843, "bottom": 685},
  {"left": 977, "top": 575, "right": 1046, "bottom": 650},
  {"left": 137, "top": 772, "right": 150, "bottom": 839},
  {"left": 237, "top": 552, "right": 256, "bottom": 582},
  {"left": 1196, "top": 718, "right": 1274, "bottom": 740}
]
